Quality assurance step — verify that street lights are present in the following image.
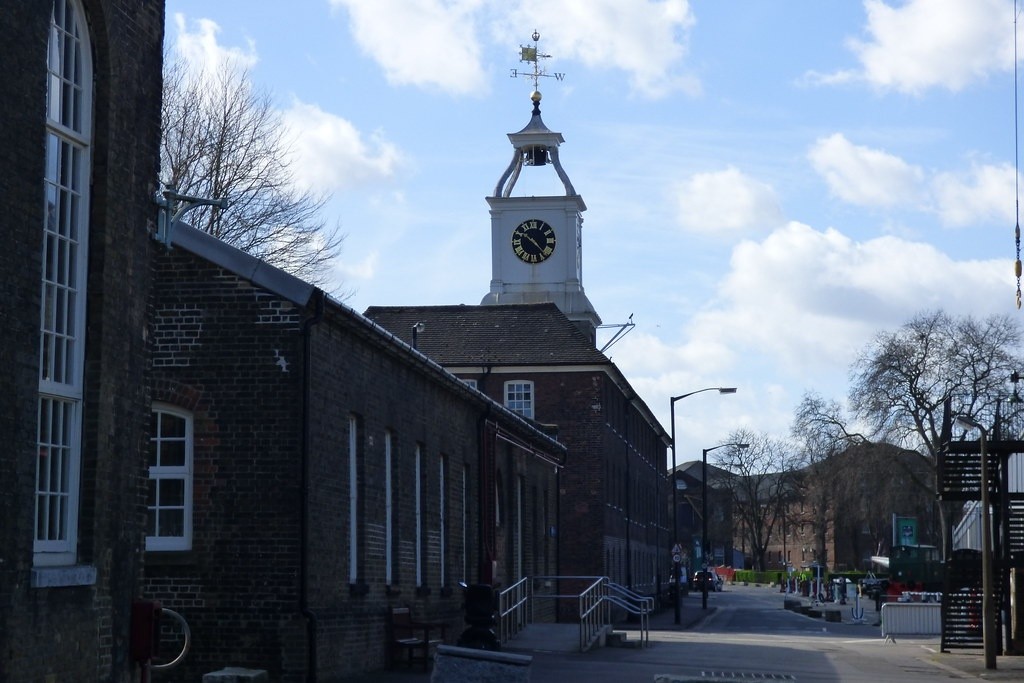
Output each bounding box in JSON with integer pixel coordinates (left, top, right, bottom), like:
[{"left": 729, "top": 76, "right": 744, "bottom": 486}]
[
  {"left": 667, "top": 385, "right": 738, "bottom": 625},
  {"left": 700, "top": 442, "right": 748, "bottom": 609}
]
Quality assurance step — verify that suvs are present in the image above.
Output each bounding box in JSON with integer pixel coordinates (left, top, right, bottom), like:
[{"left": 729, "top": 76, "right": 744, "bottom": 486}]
[{"left": 692, "top": 571, "right": 723, "bottom": 592}]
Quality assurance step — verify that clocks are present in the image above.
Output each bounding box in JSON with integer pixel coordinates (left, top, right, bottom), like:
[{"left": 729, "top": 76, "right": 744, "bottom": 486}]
[{"left": 512, "top": 218, "right": 558, "bottom": 264}]
